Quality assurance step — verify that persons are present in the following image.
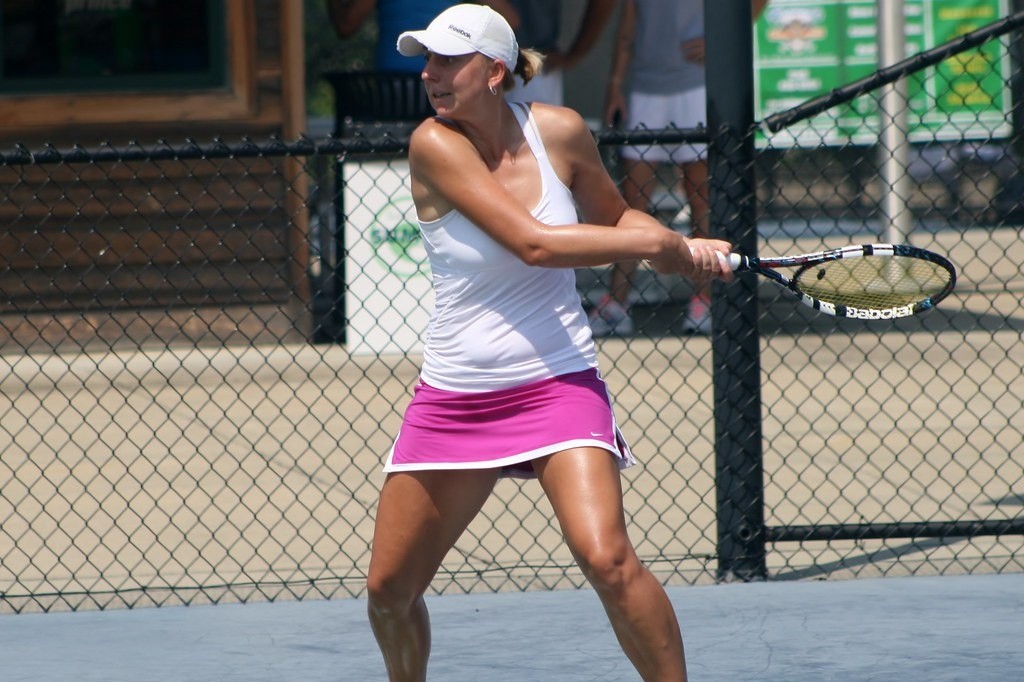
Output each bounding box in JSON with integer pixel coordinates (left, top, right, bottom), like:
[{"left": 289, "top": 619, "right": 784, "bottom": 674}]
[
  {"left": 586, "top": 0, "right": 769, "bottom": 336},
  {"left": 365, "top": 3, "right": 734, "bottom": 682},
  {"left": 325, "top": 0, "right": 614, "bottom": 106}
]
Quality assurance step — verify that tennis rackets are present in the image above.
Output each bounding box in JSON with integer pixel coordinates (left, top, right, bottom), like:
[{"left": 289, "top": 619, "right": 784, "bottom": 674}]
[{"left": 689, "top": 243, "right": 956, "bottom": 320}]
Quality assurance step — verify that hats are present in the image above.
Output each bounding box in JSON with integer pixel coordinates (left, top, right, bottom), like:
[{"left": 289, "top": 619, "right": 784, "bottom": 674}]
[{"left": 397, "top": 4, "right": 518, "bottom": 72}]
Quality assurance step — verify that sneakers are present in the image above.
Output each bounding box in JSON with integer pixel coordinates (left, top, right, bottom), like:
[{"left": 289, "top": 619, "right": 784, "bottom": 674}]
[
  {"left": 590, "top": 295, "right": 631, "bottom": 335},
  {"left": 683, "top": 295, "right": 713, "bottom": 332}
]
